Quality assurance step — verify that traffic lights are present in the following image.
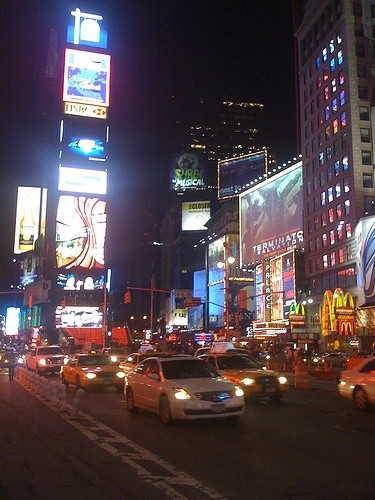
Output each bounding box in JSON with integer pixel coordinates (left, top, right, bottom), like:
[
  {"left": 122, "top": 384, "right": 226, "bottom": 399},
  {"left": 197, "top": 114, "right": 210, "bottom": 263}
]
[
  {"left": 124, "top": 293, "right": 127, "bottom": 304},
  {"left": 126, "top": 293, "right": 130, "bottom": 305}
]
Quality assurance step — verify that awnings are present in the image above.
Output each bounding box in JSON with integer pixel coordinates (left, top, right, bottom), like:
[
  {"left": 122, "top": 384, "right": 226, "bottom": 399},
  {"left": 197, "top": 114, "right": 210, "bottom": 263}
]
[{"left": 291, "top": 327, "right": 320, "bottom": 334}]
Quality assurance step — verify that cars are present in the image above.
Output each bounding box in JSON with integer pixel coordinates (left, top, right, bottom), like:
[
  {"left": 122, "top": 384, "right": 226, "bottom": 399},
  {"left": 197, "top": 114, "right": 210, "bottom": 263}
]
[
  {"left": 59, "top": 353, "right": 125, "bottom": 393},
  {"left": 123, "top": 357, "right": 246, "bottom": 426},
  {"left": 303, "top": 353, "right": 350, "bottom": 368},
  {"left": 123, "top": 353, "right": 165, "bottom": 369},
  {"left": 109, "top": 348, "right": 124, "bottom": 361},
  {"left": 25, "top": 345, "right": 67, "bottom": 375},
  {"left": 337, "top": 357, "right": 375, "bottom": 412},
  {"left": 198, "top": 353, "right": 288, "bottom": 401}
]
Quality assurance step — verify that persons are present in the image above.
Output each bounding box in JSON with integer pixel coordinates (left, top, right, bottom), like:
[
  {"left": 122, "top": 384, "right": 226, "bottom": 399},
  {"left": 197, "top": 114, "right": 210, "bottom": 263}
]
[
  {"left": 24, "top": 344, "right": 29, "bottom": 350},
  {"left": 9, "top": 361, "right": 14, "bottom": 381}
]
